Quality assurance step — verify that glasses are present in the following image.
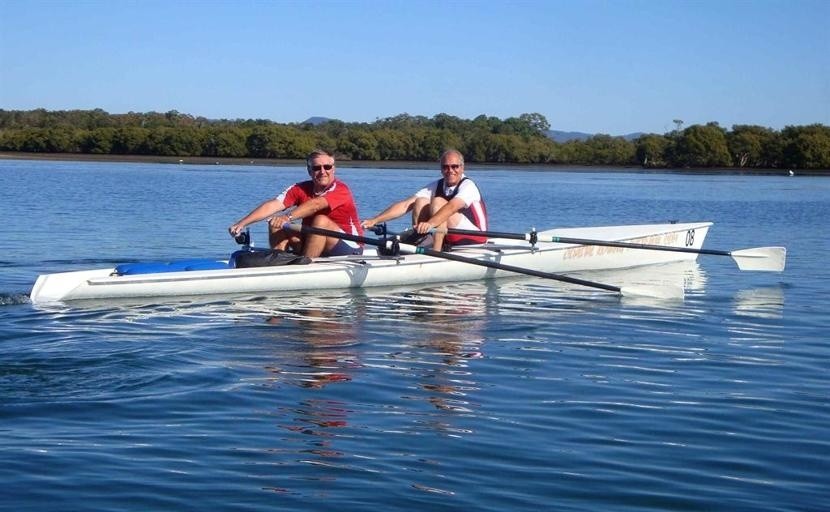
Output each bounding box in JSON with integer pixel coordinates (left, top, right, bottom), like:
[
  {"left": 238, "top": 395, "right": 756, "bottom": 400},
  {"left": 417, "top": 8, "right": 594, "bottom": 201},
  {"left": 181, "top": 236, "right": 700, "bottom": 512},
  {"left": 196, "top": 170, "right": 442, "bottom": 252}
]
[
  {"left": 440, "top": 164, "right": 460, "bottom": 169},
  {"left": 311, "top": 164, "right": 332, "bottom": 171}
]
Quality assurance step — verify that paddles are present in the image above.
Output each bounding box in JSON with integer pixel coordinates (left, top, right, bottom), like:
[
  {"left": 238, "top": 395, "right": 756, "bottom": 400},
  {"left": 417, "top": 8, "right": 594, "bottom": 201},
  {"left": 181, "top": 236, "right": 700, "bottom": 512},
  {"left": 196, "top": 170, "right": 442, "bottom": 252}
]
[
  {"left": 427, "top": 228, "right": 786, "bottom": 272},
  {"left": 284, "top": 222, "right": 684, "bottom": 300}
]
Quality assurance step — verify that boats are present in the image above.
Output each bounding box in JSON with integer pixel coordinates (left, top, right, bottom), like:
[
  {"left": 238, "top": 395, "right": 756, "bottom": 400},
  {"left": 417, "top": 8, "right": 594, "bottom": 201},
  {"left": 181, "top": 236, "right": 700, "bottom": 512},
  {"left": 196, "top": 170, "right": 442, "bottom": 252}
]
[{"left": 29, "top": 221, "right": 714, "bottom": 311}]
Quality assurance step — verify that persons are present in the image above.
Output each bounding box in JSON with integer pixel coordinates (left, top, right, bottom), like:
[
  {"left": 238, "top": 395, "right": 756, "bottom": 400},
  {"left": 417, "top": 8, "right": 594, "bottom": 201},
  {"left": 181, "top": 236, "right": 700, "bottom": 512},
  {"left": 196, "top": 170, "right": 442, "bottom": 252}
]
[
  {"left": 396, "top": 281, "right": 491, "bottom": 444},
  {"left": 362, "top": 145, "right": 488, "bottom": 255},
  {"left": 257, "top": 296, "right": 356, "bottom": 463},
  {"left": 227, "top": 148, "right": 365, "bottom": 265}
]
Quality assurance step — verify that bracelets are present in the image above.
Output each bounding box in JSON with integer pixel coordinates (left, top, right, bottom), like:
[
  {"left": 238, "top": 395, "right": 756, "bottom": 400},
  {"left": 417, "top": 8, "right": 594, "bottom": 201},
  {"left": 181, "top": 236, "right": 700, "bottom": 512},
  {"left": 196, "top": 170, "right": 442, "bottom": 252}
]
[{"left": 286, "top": 212, "right": 293, "bottom": 223}]
